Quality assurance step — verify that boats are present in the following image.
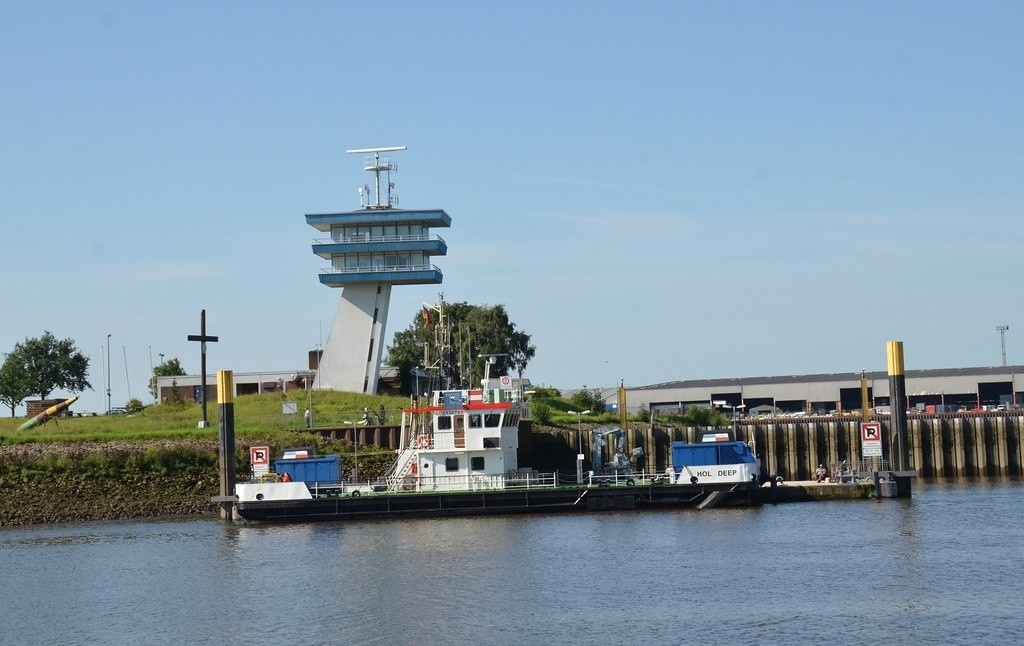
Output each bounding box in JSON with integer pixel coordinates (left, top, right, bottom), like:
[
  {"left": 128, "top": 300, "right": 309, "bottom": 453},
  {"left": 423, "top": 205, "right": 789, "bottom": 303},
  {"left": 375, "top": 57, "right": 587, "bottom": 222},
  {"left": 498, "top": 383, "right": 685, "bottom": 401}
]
[{"left": 230, "top": 355, "right": 767, "bottom": 521}]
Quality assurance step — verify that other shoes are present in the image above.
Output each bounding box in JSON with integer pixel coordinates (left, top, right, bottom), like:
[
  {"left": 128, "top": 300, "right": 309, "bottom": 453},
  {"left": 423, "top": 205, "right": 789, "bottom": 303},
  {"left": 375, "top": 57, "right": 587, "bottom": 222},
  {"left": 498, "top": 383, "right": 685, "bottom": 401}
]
[{"left": 818, "top": 480, "right": 821, "bottom": 483}]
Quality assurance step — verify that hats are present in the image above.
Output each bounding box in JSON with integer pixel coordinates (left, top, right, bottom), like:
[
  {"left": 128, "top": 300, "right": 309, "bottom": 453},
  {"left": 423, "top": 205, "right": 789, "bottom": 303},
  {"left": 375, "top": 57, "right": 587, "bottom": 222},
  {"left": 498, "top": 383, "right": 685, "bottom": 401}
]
[{"left": 820, "top": 464, "right": 822, "bottom": 466}]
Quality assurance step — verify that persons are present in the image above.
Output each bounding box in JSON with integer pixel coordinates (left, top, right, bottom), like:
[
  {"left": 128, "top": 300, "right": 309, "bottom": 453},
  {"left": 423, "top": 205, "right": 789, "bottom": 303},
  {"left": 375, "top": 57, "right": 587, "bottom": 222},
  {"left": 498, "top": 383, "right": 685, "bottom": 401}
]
[
  {"left": 840, "top": 460, "right": 847, "bottom": 472},
  {"left": 816, "top": 464, "right": 826, "bottom": 483},
  {"left": 304, "top": 407, "right": 310, "bottom": 428}
]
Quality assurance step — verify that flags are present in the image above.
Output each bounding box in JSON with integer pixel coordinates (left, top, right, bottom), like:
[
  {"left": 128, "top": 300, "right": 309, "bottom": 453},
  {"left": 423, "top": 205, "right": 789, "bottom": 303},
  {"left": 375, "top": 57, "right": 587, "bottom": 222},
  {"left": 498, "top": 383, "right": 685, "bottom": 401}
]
[{"left": 423, "top": 301, "right": 432, "bottom": 328}]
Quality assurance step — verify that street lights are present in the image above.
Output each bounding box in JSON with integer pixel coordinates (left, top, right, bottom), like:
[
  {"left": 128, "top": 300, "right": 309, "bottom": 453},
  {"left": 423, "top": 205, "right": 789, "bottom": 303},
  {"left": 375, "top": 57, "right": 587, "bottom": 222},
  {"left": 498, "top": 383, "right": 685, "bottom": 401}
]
[
  {"left": 107, "top": 334, "right": 112, "bottom": 416},
  {"left": 567, "top": 409, "right": 591, "bottom": 484}
]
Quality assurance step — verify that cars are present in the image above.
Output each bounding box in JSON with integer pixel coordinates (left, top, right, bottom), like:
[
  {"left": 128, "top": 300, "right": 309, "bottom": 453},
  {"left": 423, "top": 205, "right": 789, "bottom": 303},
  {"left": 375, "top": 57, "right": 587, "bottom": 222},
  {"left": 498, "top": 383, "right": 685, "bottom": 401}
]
[{"left": 105, "top": 409, "right": 130, "bottom": 414}]
